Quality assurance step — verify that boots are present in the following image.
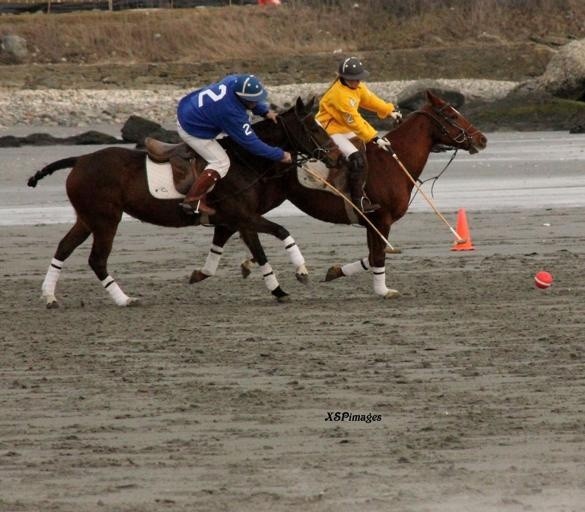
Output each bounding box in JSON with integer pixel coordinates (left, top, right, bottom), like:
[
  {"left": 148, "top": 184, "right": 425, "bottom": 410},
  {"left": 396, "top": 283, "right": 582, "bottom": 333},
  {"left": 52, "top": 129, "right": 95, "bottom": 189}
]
[
  {"left": 178, "top": 168, "right": 222, "bottom": 216},
  {"left": 346, "top": 150, "right": 382, "bottom": 215}
]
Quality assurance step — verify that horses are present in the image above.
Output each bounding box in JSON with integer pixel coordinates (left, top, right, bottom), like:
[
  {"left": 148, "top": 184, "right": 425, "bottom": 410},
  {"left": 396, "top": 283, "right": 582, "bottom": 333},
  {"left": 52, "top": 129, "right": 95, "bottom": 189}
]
[
  {"left": 188, "top": 89, "right": 488, "bottom": 300},
  {"left": 27, "top": 96, "right": 346, "bottom": 309}
]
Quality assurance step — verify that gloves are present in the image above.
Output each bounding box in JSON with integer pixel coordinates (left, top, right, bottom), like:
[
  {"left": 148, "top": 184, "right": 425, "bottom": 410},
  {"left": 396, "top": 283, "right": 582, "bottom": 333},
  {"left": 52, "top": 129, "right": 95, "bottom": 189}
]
[
  {"left": 375, "top": 137, "right": 390, "bottom": 153},
  {"left": 390, "top": 110, "right": 403, "bottom": 123}
]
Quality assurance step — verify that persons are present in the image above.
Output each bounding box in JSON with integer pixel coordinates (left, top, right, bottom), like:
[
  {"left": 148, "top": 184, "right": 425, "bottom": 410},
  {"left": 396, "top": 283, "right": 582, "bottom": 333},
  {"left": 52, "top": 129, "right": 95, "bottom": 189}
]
[
  {"left": 176, "top": 73, "right": 293, "bottom": 216},
  {"left": 314, "top": 56, "right": 403, "bottom": 215}
]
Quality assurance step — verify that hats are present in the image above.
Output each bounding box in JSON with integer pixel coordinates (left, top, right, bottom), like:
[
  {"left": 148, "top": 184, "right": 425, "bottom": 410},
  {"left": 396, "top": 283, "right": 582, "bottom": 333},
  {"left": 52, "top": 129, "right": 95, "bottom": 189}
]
[
  {"left": 338, "top": 56, "right": 370, "bottom": 81},
  {"left": 234, "top": 74, "right": 268, "bottom": 103}
]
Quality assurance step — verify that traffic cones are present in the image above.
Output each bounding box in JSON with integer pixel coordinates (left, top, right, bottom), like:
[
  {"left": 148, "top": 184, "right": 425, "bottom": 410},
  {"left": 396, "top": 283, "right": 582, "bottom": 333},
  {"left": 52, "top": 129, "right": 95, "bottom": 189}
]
[{"left": 450, "top": 209, "right": 476, "bottom": 252}]
